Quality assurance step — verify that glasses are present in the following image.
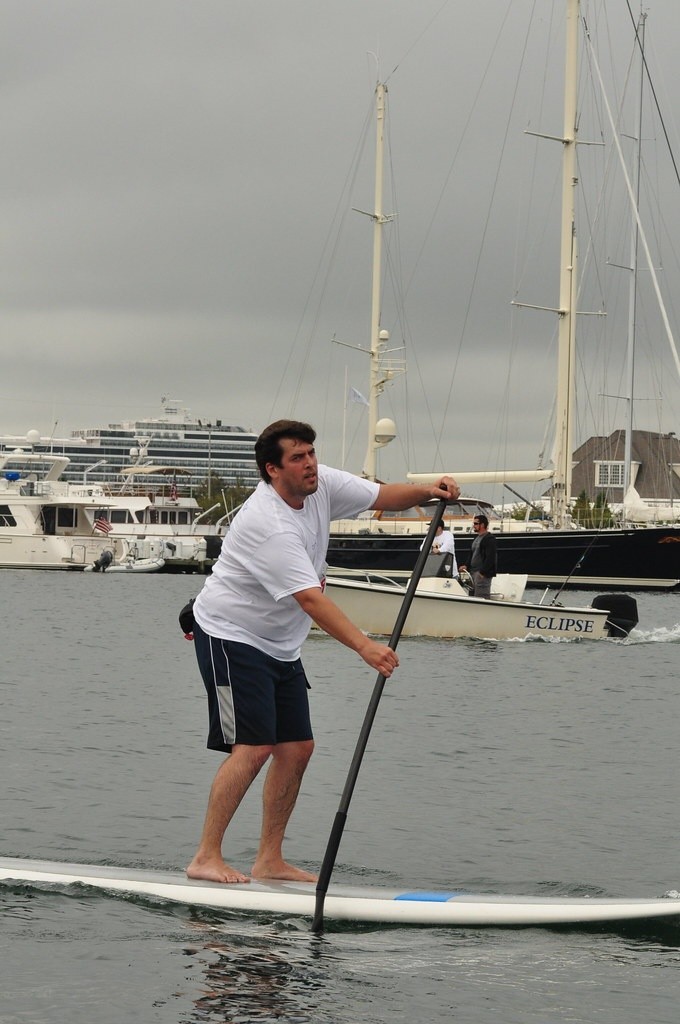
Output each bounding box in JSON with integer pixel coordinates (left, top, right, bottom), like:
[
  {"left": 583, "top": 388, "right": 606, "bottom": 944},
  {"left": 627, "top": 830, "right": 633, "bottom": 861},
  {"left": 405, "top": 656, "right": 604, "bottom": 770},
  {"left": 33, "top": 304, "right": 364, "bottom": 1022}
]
[{"left": 474, "top": 522, "right": 481, "bottom": 525}]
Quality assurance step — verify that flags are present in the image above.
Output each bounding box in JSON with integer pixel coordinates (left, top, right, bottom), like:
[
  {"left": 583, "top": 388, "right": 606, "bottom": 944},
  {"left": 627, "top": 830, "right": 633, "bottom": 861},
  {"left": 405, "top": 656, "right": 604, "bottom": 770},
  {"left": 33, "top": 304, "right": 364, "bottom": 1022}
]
[
  {"left": 95, "top": 516, "right": 113, "bottom": 533},
  {"left": 171, "top": 473, "right": 178, "bottom": 501}
]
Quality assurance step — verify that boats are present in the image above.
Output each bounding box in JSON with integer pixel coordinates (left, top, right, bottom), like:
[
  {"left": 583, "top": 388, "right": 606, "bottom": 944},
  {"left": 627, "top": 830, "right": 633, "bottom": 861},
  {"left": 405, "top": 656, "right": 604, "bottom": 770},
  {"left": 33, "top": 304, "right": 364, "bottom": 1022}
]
[
  {"left": 320, "top": 563, "right": 527, "bottom": 601},
  {"left": 97, "top": 440, "right": 235, "bottom": 572},
  {"left": 0, "top": 447, "right": 164, "bottom": 575},
  {"left": 318, "top": 0, "right": 680, "bottom": 593},
  {"left": 310, "top": 576, "right": 611, "bottom": 641},
  {"left": 0, "top": 858, "right": 680, "bottom": 933}
]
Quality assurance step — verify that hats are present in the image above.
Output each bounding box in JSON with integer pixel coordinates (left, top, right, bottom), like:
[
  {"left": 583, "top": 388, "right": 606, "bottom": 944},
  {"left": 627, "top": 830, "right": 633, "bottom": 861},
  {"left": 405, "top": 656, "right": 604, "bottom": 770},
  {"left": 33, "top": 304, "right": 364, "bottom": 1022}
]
[{"left": 426, "top": 519, "right": 444, "bottom": 528}]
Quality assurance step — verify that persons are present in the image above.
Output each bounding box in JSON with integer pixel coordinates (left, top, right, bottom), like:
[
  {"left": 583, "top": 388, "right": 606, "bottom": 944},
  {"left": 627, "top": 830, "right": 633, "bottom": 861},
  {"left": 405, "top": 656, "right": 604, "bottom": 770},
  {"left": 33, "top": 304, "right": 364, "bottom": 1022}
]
[
  {"left": 461, "top": 515, "right": 496, "bottom": 600},
  {"left": 189, "top": 421, "right": 460, "bottom": 883},
  {"left": 420, "top": 518, "right": 459, "bottom": 580}
]
